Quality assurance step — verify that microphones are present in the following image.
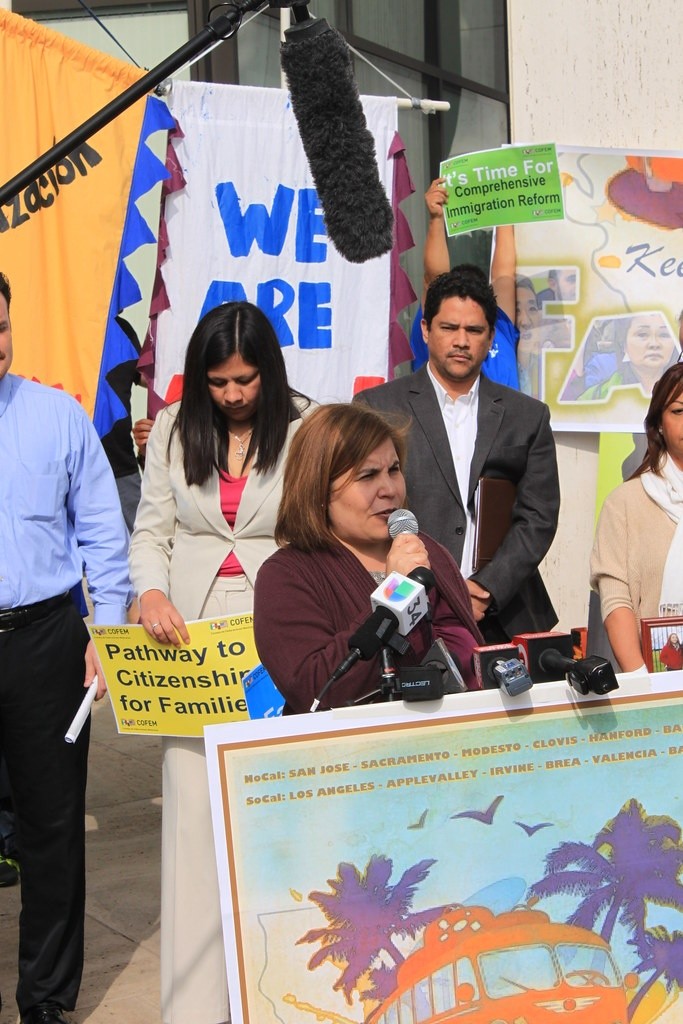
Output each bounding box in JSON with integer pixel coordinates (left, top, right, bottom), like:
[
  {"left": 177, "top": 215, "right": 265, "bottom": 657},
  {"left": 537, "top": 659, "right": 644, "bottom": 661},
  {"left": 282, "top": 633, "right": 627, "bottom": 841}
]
[
  {"left": 399, "top": 638, "right": 468, "bottom": 702},
  {"left": 388, "top": 509, "right": 419, "bottom": 538},
  {"left": 470, "top": 643, "right": 533, "bottom": 697},
  {"left": 333, "top": 567, "right": 435, "bottom": 679},
  {"left": 512, "top": 632, "right": 620, "bottom": 696}
]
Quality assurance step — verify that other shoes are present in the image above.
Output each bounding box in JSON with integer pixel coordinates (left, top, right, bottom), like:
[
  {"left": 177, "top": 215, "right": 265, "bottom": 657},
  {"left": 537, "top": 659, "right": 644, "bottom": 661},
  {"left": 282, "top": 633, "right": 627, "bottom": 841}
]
[
  {"left": 0, "top": 845, "right": 20, "bottom": 889},
  {"left": 18, "top": 1000, "right": 67, "bottom": 1024}
]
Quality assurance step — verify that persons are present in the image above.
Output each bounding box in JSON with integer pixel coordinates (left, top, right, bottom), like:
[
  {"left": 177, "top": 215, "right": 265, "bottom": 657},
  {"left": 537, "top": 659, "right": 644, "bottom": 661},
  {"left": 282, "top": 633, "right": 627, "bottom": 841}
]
[
  {"left": 0, "top": 275, "right": 134, "bottom": 1023},
  {"left": 590, "top": 361, "right": 683, "bottom": 676},
  {"left": 254, "top": 404, "right": 487, "bottom": 718},
  {"left": 408, "top": 177, "right": 520, "bottom": 392},
  {"left": 128, "top": 302, "right": 321, "bottom": 1024},
  {"left": 535, "top": 268, "right": 576, "bottom": 308},
  {"left": 352, "top": 264, "right": 561, "bottom": 646},
  {"left": 514, "top": 269, "right": 540, "bottom": 397},
  {"left": 576, "top": 313, "right": 683, "bottom": 403}
]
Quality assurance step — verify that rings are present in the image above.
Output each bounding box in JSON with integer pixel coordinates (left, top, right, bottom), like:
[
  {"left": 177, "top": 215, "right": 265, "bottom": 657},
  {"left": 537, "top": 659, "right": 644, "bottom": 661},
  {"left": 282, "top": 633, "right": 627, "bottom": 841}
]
[{"left": 151, "top": 624, "right": 160, "bottom": 629}]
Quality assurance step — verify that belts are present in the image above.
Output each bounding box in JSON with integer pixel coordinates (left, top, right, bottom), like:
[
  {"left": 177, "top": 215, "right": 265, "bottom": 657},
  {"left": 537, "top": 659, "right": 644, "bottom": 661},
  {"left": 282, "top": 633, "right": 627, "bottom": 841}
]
[{"left": 0, "top": 590, "right": 73, "bottom": 633}]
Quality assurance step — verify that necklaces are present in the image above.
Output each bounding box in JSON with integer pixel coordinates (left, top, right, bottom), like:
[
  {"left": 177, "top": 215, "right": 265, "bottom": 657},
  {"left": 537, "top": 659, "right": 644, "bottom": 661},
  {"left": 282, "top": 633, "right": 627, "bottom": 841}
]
[{"left": 226, "top": 428, "right": 252, "bottom": 458}]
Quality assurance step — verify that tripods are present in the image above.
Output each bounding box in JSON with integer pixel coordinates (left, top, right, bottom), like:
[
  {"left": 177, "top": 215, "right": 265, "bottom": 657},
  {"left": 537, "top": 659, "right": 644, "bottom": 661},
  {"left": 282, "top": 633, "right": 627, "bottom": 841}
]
[{"left": 343, "top": 646, "right": 400, "bottom": 708}]
